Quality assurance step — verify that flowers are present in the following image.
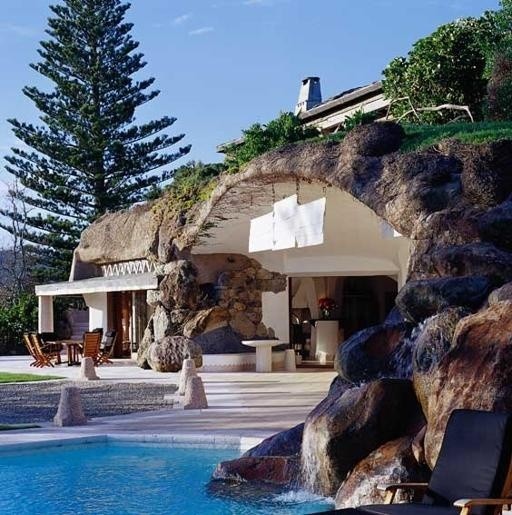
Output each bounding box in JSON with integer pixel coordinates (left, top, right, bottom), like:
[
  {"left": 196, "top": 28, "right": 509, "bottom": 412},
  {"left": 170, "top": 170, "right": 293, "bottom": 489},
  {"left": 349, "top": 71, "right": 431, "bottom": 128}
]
[{"left": 319, "top": 297, "right": 338, "bottom": 313}]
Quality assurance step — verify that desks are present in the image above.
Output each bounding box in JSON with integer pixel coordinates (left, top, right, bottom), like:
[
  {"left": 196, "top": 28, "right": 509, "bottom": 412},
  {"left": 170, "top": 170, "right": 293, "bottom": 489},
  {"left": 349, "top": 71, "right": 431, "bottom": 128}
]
[{"left": 241, "top": 340, "right": 279, "bottom": 373}]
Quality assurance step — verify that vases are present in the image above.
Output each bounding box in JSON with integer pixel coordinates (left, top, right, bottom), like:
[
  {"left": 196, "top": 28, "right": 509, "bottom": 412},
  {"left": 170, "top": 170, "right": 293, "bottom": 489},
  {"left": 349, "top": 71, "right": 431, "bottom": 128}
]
[{"left": 324, "top": 309, "right": 330, "bottom": 320}]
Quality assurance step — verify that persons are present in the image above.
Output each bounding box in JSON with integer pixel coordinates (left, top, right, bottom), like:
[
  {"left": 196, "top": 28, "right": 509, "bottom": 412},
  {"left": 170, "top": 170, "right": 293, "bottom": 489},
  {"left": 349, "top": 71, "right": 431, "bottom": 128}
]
[
  {"left": 64, "top": 323, "right": 72, "bottom": 339},
  {"left": 56, "top": 321, "right": 65, "bottom": 340}
]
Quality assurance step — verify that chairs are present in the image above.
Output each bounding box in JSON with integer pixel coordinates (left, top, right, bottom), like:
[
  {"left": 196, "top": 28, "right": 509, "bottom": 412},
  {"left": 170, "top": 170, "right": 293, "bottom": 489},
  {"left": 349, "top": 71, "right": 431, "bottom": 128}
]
[
  {"left": 22, "top": 328, "right": 119, "bottom": 369},
  {"left": 356, "top": 408, "right": 512, "bottom": 514}
]
